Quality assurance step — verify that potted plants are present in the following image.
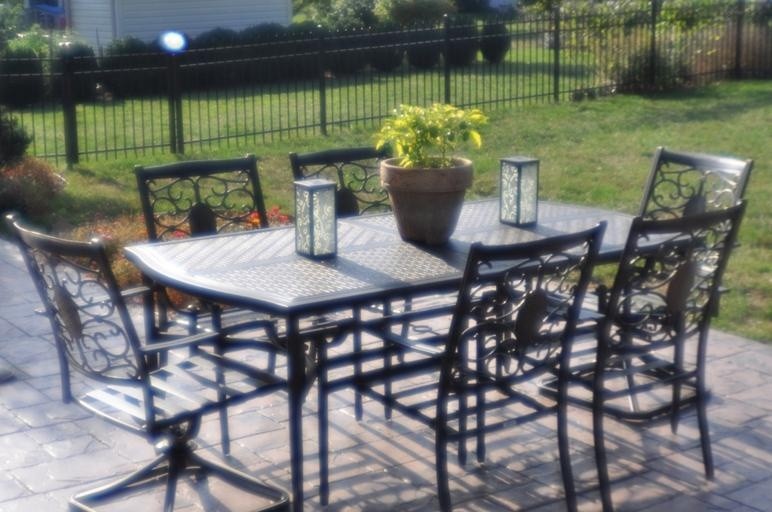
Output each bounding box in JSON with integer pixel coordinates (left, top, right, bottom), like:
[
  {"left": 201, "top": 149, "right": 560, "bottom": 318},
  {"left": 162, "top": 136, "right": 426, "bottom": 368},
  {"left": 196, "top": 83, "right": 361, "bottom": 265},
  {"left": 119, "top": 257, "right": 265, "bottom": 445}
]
[{"left": 369, "top": 100, "right": 491, "bottom": 246}]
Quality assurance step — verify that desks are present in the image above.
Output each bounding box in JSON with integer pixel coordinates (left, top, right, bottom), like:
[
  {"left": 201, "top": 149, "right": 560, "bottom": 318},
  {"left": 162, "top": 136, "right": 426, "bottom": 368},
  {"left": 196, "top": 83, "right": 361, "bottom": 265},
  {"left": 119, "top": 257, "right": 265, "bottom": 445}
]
[{"left": 121, "top": 195, "right": 713, "bottom": 510}]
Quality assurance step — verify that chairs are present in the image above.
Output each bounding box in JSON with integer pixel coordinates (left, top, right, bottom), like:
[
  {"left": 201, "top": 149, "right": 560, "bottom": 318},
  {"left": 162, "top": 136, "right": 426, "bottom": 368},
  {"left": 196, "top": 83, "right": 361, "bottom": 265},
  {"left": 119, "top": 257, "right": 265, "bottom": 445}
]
[
  {"left": 324, "top": 219, "right": 608, "bottom": 512},
  {"left": 283, "top": 142, "right": 410, "bottom": 423},
  {"left": 6, "top": 215, "right": 296, "bottom": 512},
  {"left": 547, "top": 146, "right": 755, "bottom": 419},
  {"left": 512, "top": 201, "right": 745, "bottom": 508},
  {"left": 128, "top": 154, "right": 353, "bottom": 411}
]
